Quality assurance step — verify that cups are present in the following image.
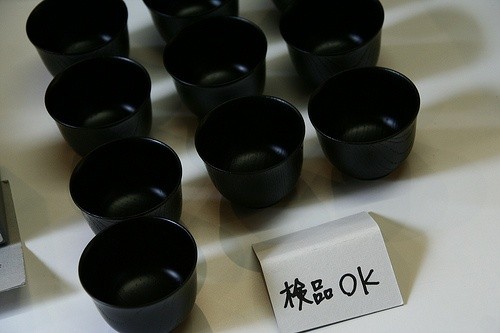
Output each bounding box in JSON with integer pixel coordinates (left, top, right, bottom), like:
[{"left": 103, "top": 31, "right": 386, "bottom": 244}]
[
  {"left": 25, "top": 0, "right": 129, "bottom": 77},
  {"left": 44, "top": 57, "right": 152, "bottom": 155},
  {"left": 162, "top": 17, "right": 268, "bottom": 115},
  {"left": 273, "top": 0, "right": 385, "bottom": 88},
  {"left": 194, "top": 95, "right": 305, "bottom": 206},
  {"left": 69, "top": 136, "right": 183, "bottom": 235},
  {"left": 143, "top": 0, "right": 240, "bottom": 42},
  {"left": 79, "top": 220, "right": 198, "bottom": 333},
  {"left": 306, "top": 66, "right": 421, "bottom": 180}
]
